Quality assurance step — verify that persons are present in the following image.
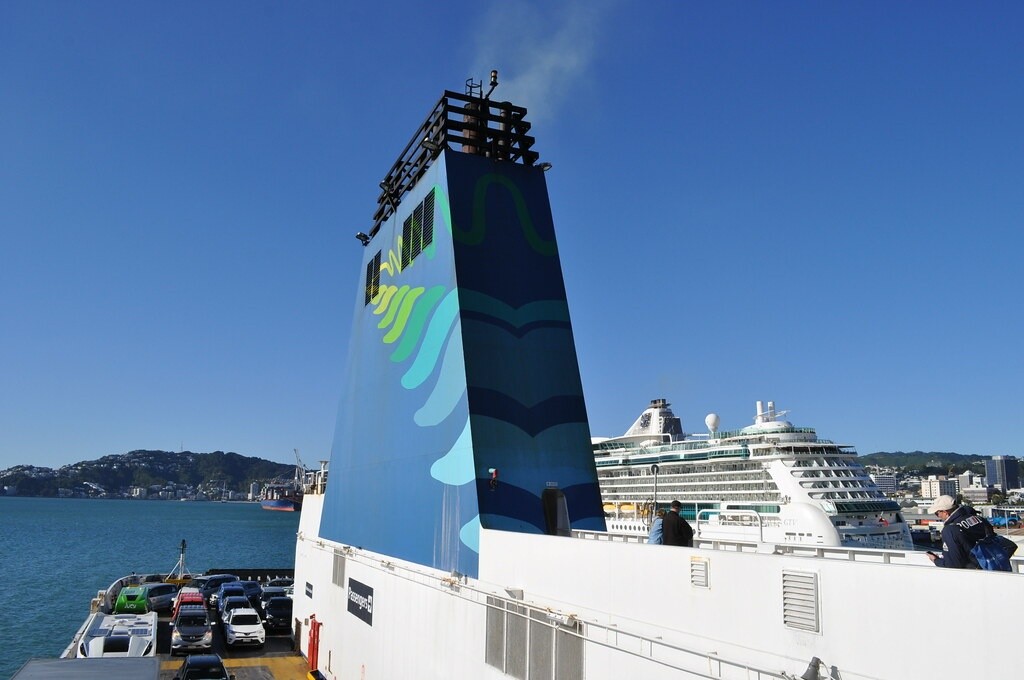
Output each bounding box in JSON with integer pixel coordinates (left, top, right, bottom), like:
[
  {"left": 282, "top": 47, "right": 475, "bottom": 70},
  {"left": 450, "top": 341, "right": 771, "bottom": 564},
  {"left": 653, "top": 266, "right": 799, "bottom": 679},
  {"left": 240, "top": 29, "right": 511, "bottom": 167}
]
[
  {"left": 878, "top": 516, "right": 889, "bottom": 527},
  {"left": 650, "top": 508, "right": 666, "bottom": 547},
  {"left": 663, "top": 499, "right": 695, "bottom": 547}
]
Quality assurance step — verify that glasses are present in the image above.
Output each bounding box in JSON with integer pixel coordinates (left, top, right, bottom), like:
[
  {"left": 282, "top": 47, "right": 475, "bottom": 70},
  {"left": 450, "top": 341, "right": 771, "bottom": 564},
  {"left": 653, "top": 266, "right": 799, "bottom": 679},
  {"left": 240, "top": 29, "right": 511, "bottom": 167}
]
[{"left": 935, "top": 511, "right": 941, "bottom": 516}]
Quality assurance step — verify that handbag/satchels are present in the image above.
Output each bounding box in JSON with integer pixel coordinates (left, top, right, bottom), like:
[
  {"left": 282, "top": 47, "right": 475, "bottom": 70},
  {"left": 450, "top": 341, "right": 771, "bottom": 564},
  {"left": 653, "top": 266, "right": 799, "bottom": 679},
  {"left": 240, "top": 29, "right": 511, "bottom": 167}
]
[
  {"left": 971, "top": 537, "right": 1013, "bottom": 571},
  {"left": 972, "top": 534, "right": 1018, "bottom": 569}
]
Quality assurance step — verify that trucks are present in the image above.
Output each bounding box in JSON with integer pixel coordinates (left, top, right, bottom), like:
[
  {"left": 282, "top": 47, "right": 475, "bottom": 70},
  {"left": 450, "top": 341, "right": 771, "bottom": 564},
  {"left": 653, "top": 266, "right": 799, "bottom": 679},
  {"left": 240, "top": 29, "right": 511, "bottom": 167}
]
[{"left": 77, "top": 612, "right": 158, "bottom": 658}]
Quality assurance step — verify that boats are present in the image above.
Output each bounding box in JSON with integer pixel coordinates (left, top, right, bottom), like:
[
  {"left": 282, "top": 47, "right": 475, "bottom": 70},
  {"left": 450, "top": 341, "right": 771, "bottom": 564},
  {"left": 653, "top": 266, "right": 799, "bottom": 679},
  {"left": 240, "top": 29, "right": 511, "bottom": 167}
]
[
  {"left": 602, "top": 504, "right": 616, "bottom": 515},
  {"left": 620, "top": 505, "right": 640, "bottom": 514},
  {"left": 261, "top": 499, "right": 302, "bottom": 512}
]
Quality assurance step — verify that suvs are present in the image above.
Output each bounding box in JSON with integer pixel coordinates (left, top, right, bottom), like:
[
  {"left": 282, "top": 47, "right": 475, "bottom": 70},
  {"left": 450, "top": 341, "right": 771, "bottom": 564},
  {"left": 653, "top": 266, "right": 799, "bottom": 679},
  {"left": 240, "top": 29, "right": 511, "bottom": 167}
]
[
  {"left": 169, "top": 586, "right": 217, "bottom": 658},
  {"left": 219, "top": 582, "right": 253, "bottom": 624},
  {"left": 224, "top": 607, "right": 267, "bottom": 651},
  {"left": 264, "top": 597, "right": 293, "bottom": 634},
  {"left": 208, "top": 581, "right": 263, "bottom": 607},
  {"left": 172, "top": 652, "right": 235, "bottom": 680}
]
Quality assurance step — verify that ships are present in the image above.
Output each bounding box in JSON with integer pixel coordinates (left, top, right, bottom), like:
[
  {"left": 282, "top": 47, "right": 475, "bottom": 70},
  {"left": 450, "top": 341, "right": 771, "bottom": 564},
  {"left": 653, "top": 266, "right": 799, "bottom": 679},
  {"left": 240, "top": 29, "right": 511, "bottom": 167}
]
[{"left": 592, "top": 399, "right": 915, "bottom": 551}]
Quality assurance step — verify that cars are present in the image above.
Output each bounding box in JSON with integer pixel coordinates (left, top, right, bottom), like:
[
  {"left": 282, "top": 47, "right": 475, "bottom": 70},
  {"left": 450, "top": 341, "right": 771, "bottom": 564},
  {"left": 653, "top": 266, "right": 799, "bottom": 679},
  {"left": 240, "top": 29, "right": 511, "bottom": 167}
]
[
  {"left": 138, "top": 583, "right": 178, "bottom": 615},
  {"left": 260, "top": 577, "right": 295, "bottom": 610}
]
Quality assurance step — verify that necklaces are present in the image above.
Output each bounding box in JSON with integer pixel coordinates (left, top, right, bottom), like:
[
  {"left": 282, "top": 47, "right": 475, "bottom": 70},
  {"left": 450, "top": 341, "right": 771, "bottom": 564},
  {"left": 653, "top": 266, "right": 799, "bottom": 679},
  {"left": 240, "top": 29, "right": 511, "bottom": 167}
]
[{"left": 923, "top": 495, "right": 1018, "bottom": 572}]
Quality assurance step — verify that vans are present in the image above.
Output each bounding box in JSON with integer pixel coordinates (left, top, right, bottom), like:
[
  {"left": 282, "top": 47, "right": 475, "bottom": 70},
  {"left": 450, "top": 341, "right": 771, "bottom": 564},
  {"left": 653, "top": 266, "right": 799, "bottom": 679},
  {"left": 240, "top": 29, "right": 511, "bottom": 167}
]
[{"left": 183, "top": 574, "right": 237, "bottom": 605}]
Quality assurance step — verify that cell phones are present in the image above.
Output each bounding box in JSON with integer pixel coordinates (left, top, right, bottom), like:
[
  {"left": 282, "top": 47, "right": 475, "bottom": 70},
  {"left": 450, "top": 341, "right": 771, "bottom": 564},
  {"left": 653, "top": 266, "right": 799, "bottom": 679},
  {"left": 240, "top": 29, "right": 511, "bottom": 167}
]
[{"left": 927, "top": 551, "right": 938, "bottom": 558}]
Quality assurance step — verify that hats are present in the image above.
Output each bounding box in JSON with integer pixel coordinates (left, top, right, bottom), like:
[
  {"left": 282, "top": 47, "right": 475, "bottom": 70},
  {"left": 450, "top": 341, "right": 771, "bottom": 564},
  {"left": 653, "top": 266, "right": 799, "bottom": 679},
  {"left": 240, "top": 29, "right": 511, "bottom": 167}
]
[
  {"left": 927, "top": 495, "right": 957, "bottom": 514},
  {"left": 671, "top": 500, "right": 681, "bottom": 509}
]
